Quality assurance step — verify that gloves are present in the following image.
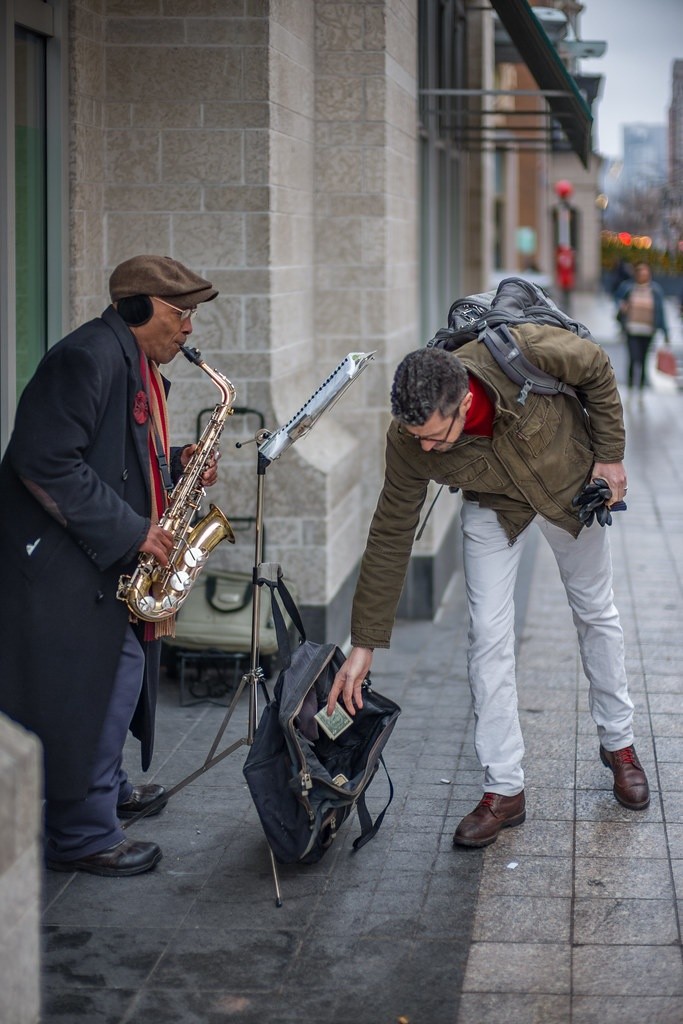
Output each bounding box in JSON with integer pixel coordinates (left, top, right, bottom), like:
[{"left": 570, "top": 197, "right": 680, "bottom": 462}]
[{"left": 572, "top": 477, "right": 627, "bottom": 528}]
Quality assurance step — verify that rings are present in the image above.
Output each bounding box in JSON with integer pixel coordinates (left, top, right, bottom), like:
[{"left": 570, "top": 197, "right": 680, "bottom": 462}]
[{"left": 623, "top": 487, "right": 629, "bottom": 490}]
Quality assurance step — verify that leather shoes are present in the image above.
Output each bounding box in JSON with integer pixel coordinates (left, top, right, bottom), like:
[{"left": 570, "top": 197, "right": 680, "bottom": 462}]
[
  {"left": 453, "top": 789, "right": 525, "bottom": 847},
  {"left": 115, "top": 784, "right": 167, "bottom": 818},
  {"left": 599, "top": 743, "right": 651, "bottom": 811},
  {"left": 46, "top": 836, "right": 163, "bottom": 875}
]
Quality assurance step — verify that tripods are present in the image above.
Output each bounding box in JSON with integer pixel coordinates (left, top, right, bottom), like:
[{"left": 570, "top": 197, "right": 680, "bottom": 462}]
[{"left": 122, "top": 350, "right": 377, "bottom": 909}]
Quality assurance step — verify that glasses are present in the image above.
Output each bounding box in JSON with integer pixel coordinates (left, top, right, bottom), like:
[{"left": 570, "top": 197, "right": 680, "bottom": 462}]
[
  {"left": 151, "top": 294, "right": 198, "bottom": 322},
  {"left": 397, "top": 406, "right": 458, "bottom": 446}
]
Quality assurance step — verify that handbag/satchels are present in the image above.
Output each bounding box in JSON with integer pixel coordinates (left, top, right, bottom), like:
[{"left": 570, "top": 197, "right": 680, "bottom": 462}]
[
  {"left": 657, "top": 346, "right": 676, "bottom": 374},
  {"left": 241, "top": 563, "right": 404, "bottom": 867}
]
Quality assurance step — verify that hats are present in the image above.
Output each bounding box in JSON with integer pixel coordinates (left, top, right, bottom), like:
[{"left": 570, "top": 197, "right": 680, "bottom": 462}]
[{"left": 108, "top": 255, "right": 220, "bottom": 309}]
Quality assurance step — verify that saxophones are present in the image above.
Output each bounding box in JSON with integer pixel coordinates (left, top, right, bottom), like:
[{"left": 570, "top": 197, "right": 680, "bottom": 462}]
[{"left": 115, "top": 345, "right": 237, "bottom": 624}]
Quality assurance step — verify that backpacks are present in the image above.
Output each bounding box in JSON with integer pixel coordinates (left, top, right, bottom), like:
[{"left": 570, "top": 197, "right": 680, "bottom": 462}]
[{"left": 424, "top": 276, "right": 597, "bottom": 420}]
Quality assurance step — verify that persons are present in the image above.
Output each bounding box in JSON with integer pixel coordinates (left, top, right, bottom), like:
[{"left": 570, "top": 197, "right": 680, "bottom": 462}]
[
  {"left": 0, "top": 253, "right": 223, "bottom": 876},
  {"left": 604, "top": 254, "right": 672, "bottom": 400},
  {"left": 327, "top": 321, "right": 651, "bottom": 847}
]
